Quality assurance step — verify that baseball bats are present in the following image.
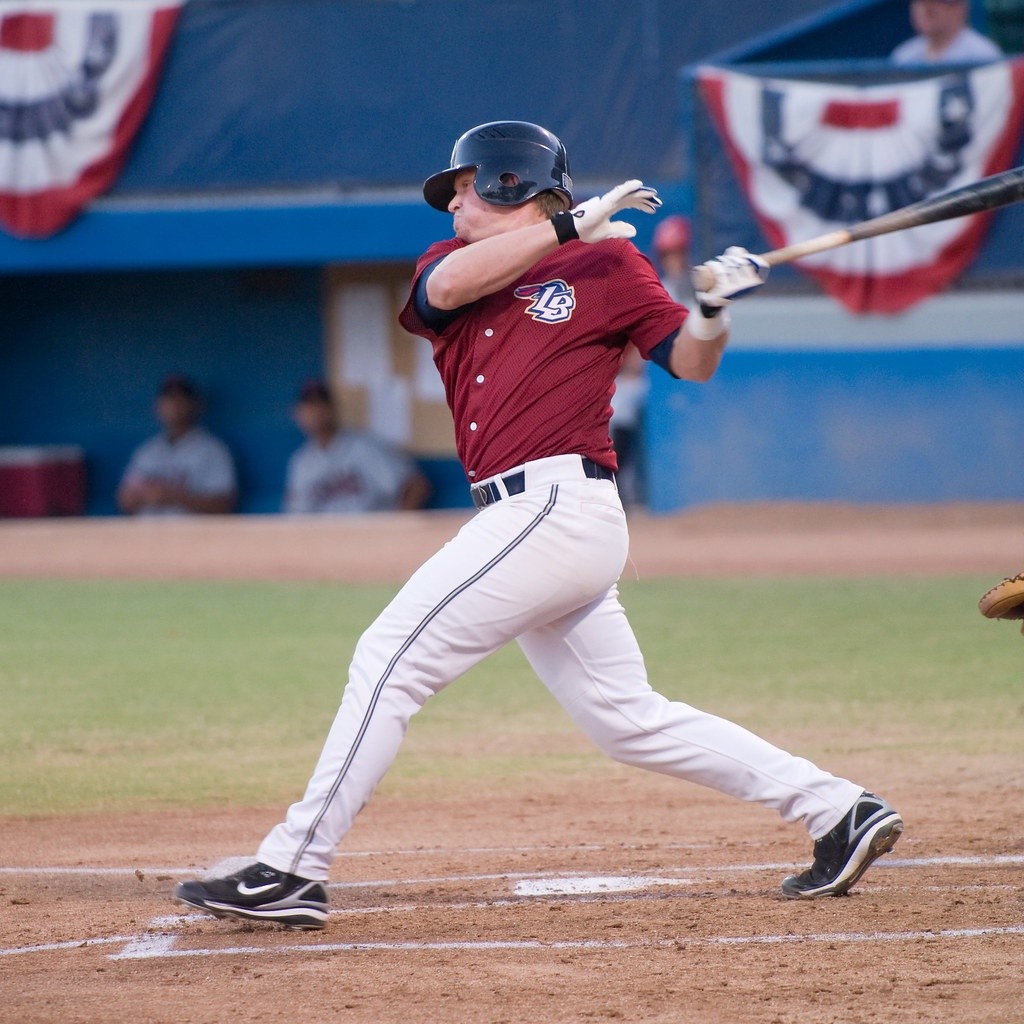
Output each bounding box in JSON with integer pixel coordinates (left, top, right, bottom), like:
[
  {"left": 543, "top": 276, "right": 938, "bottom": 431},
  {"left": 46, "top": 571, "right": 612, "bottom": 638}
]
[{"left": 687, "top": 165, "right": 1024, "bottom": 293}]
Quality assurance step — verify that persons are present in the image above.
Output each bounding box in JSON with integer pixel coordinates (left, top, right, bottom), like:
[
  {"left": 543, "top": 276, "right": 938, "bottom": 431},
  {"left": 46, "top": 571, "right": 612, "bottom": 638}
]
[
  {"left": 891, "top": 0, "right": 1005, "bottom": 68},
  {"left": 169, "top": 121, "right": 903, "bottom": 930},
  {"left": 286, "top": 374, "right": 424, "bottom": 515},
  {"left": 119, "top": 374, "right": 235, "bottom": 517}
]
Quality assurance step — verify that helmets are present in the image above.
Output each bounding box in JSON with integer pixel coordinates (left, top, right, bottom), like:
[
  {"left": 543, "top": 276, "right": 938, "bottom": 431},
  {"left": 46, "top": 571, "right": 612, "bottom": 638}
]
[{"left": 423, "top": 121, "right": 573, "bottom": 212}]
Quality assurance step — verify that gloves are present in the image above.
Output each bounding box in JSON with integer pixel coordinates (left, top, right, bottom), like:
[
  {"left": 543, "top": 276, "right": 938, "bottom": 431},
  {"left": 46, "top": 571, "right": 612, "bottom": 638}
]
[
  {"left": 550, "top": 179, "right": 663, "bottom": 246},
  {"left": 695, "top": 246, "right": 769, "bottom": 318}
]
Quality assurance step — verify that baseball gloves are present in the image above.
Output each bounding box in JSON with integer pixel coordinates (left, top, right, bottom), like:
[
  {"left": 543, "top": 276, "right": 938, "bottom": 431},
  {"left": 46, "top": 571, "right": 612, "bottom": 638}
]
[{"left": 977, "top": 572, "right": 1024, "bottom": 636}]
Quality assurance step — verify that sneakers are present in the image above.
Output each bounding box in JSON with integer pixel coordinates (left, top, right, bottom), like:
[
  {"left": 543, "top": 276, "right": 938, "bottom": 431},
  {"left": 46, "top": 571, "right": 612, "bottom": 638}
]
[
  {"left": 780, "top": 791, "right": 904, "bottom": 897},
  {"left": 172, "top": 862, "right": 330, "bottom": 930}
]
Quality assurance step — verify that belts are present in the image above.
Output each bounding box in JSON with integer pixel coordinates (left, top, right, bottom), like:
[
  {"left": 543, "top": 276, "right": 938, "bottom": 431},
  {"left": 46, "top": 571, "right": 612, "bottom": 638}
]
[{"left": 469, "top": 458, "right": 614, "bottom": 509}]
